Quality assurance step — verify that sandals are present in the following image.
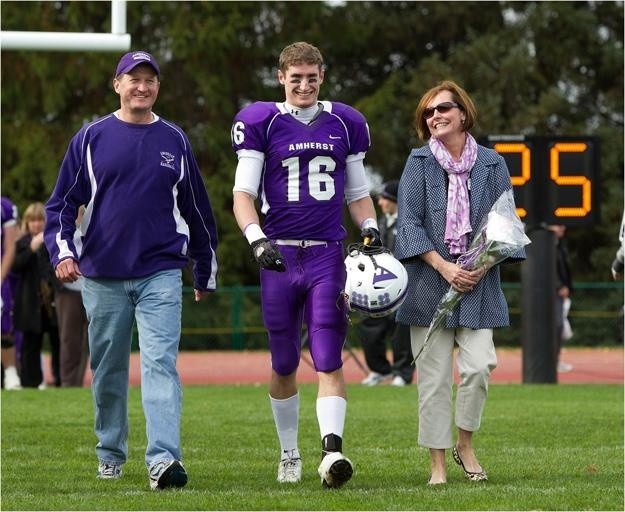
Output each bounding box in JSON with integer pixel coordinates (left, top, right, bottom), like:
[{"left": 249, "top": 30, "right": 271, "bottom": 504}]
[
  {"left": 428, "top": 476, "right": 445, "bottom": 486},
  {"left": 454, "top": 446, "right": 486, "bottom": 481}
]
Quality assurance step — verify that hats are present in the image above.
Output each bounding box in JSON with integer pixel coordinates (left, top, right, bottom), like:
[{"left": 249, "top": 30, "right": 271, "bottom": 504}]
[
  {"left": 380, "top": 184, "right": 399, "bottom": 203},
  {"left": 116, "top": 53, "right": 160, "bottom": 77}
]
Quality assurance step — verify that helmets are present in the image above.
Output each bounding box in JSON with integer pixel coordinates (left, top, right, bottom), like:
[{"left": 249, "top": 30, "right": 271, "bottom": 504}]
[{"left": 343, "top": 247, "right": 408, "bottom": 319}]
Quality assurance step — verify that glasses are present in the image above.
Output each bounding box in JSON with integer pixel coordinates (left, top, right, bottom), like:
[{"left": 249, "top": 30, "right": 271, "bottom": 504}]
[{"left": 423, "top": 101, "right": 459, "bottom": 119}]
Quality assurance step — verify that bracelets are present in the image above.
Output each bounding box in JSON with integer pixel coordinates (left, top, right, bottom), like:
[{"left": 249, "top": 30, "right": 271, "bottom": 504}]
[{"left": 481, "top": 263, "right": 488, "bottom": 276}]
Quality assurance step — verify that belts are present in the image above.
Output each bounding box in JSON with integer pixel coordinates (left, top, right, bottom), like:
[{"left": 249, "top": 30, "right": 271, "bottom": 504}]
[{"left": 274, "top": 239, "right": 337, "bottom": 248}]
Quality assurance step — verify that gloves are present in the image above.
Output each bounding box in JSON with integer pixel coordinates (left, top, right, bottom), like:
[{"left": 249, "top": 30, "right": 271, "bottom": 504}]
[
  {"left": 253, "top": 241, "right": 286, "bottom": 272},
  {"left": 361, "top": 229, "right": 384, "bottom": 254}
]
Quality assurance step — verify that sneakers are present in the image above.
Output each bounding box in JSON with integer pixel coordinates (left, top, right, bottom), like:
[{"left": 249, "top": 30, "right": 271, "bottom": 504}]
[
  {"left": 392, "top": 377, "right": 404, "bottom": 386},
  {"left": 5, "top": 370, "right": 20, "bottom": 388},
  {"left": 318, "top": 454, "right": 352, "bottom": 489},
  {"left": 149, "top": 460, "right": 187, "bottom": 491},
  {"left": 278, "top": 451, "right": 299, "bottom": 484},
  {"left": 362, "top": 370, "right": 393, "bottom": 387},
  {"left": 97, "top": 462, "right": 122, "bottom": 480}
]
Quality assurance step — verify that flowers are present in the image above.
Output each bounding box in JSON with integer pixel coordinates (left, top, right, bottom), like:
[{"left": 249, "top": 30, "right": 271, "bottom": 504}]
[{"left": 410, "top": 189, "right": 532, "bottom": 366}]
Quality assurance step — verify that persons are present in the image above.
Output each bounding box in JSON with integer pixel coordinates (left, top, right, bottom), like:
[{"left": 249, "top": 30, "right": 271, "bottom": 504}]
[
  {"left": 232, "top": 42, "right": 381, "bottom": 487},
  {"left": 546, "top": 216, "right": 570, "bottom": 375},
  {"left": 42, "top": 52, "right": 218, "bottom": 491},
  {"left": 2, "top": 196, "right": 87, "bottom": 388},
  {"left": 362, "top": 183, "right": 415, "bottom": 388},
  {"left": 397, "top": 82, "right": 528, "bottom": 489}
]
